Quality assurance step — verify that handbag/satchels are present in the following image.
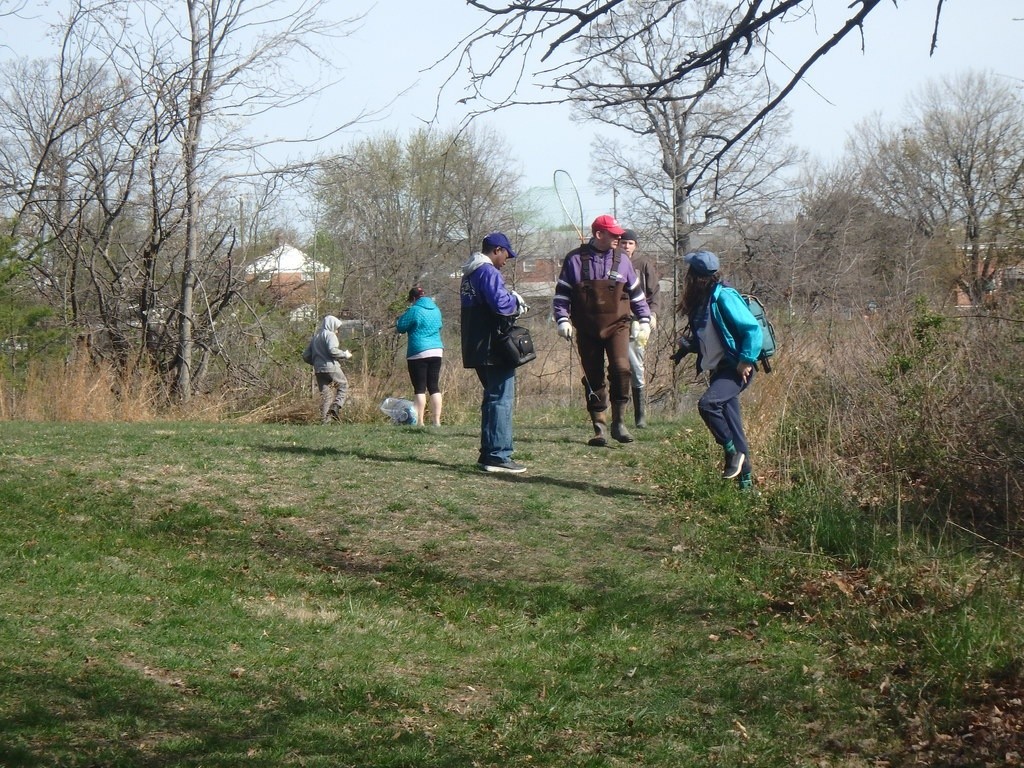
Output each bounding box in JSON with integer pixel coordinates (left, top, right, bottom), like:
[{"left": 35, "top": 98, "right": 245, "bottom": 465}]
[{"left": 495, "top": 326, "right": 536, "bottom": 369}]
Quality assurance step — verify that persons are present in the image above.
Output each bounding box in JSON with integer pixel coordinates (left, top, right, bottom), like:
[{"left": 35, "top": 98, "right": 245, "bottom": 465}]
[
  {"left": 617, "top": 229, "right": 660, "bottom": 429},
  {"left": 302, "top": 314, "right": 352, "bottom": 424},
  {"left": 397, "top": 287, "right": 444, "bottom": 430},
  {"left": 552, "top": 216, "right": 651, "bottom": 445},
  {"left": 460, "top": 233, "right": 530, "bottom": 475},
  {"left": 683, "top": 250, "right": 763, "bottom": 497}
]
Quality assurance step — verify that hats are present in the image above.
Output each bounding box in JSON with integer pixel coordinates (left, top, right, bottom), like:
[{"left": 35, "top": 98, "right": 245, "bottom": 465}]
[
  {"left": 621, "top": 229, "right": 637, "bottom": 242},
  {"left": 406, "top": 287, "right": 424, "bottom": 301},
  {"left": 684, "top": 251, "right": 719, "bottom": 275},
  {"left": 592, "top": 215, "right": 625, "bottom": 235},
  {"left": 483, "top": 232, "right": 517, "bottom": 258}
]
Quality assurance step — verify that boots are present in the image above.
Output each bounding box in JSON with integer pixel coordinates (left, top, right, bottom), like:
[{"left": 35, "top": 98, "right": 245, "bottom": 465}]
[
  {"left": 331, "top": 404, "right": 341, "bottom": 421},
  {"left": 612, "top": 400, "right": 633, "bottom": 442},
  {"left": 589, "top": 409, "right": 608, "bottom": 444},
  {"left": 632, "top": 386, "right": 646, "bottom": 428}
]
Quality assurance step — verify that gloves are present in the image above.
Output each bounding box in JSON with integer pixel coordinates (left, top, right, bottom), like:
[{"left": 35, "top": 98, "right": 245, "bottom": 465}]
[
  {"left": 556, "top": 317, "right": 572, "bottom": 341},
  {"left": 345, "top": 350, "right": 352, "bottom": 358},
  {"left": 650, "top": 312, "right": 656, "bottom": 331},
  {"left": 518, "top": 304, "right": 530, "bottom": 314},
  {"left": 511, "top": 290, "right": 524, "bottom": 304},
  {"left": 639, "top": 318, "right": 651, "bottom": 339}
]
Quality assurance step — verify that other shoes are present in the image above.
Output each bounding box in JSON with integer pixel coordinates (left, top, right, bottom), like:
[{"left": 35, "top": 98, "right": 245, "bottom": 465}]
[
  {"left": 433, "top": 423, "right": 441, "bottom": 426},
  {"left": 740, "top": 473, "right": 752, "bottom": 487},
  {"left": 723, "top": 453, "right": 745, "bottom": 479},
  {"left": 418, "top": 424, "right": 424, "bottom": 427}
]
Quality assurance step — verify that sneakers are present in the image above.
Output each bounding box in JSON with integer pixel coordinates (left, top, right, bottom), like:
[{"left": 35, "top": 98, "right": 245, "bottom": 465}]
[
  {"left": 477, "top": 453, "right": 487, "bottom": 466},
  {"left": 484, "top": 462, "right": 526, "bottom": 472}
]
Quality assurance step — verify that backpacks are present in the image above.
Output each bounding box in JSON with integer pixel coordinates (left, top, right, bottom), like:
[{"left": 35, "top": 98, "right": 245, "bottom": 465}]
[{"left": 741, "top": 294, "right": 776, "bottom": 357}]
